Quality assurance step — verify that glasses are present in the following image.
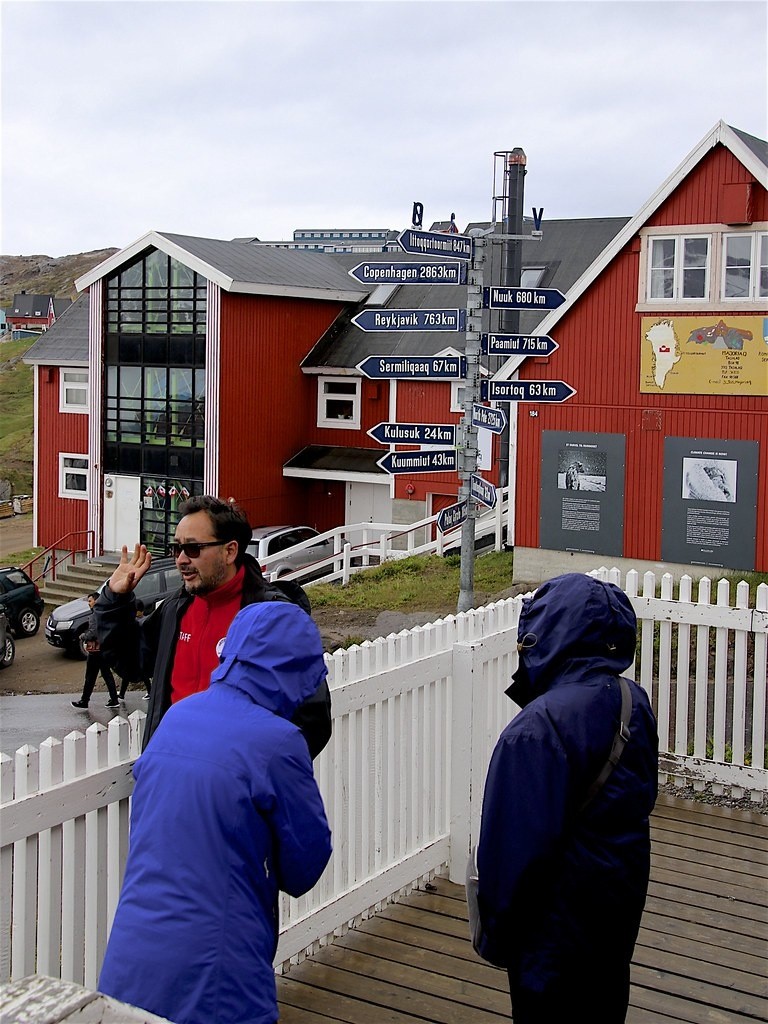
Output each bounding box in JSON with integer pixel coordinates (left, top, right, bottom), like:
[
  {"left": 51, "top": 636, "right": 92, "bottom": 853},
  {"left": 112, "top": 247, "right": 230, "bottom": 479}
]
[{"left": 168, "top": 541, "right": 227, "bottom": 558}]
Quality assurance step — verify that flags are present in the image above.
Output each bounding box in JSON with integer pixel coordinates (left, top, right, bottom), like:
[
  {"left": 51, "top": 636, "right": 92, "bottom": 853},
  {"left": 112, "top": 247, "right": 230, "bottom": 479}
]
[{"left": 145, "top": 485, "right": 190, "bottom": 499}]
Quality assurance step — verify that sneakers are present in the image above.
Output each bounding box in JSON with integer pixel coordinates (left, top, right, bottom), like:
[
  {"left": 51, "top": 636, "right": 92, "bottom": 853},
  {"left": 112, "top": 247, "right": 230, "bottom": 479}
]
[
  {"left": 117, "top": 694, "right": 125, "bottom": 700},
  {"left": 105, "top": 701, "right": 121, "bottom": 708},
  {"left": 70, "top": 700, "right": 88, "bottom": 710},
  {"left": 142, "top": 693, "right": 150, "bottom": 699}
]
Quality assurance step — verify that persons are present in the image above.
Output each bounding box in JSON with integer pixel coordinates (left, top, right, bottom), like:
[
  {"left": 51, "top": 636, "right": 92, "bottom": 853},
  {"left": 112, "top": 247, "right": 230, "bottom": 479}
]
[
  {"left": 70, "top": 592, "right": 121, "bottom": 709},
  {"left": 96, "top": 601, "right": 334, "bottom": 1024},
  {"left": 472, "top": 572, "right": 660, "bottom": 1024},
  {"left": 117, "top": 599, "right": 152, "bottom": 700},
  {"left": 93, "top": 495, "right": 332, "bottom": 765}
]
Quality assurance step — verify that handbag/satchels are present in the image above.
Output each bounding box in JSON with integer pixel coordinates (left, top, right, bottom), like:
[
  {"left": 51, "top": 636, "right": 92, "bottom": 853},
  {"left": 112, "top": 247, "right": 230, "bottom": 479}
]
[
  {"left": 462, "top": 841, "right": 556, "bottom": 969},
  {"left": 86, "top": 639, "right": 100, "bottom": 651}
]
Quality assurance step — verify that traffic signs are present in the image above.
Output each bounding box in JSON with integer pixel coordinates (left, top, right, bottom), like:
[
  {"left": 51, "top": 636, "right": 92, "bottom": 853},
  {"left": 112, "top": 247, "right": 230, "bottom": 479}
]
[
  {"left": 470, "top": 474, "right": 497, "bottom": 510},
  {"left": 395, "top": 228, "right": 473, "bottom": 262},
  {"left": 368, "top": 423, "right": 456, "bottom": 446},
  {"left": 489, "top": 286, "right": 568, "bottom": 310},
  {"left": 487, "top": 331, "right": 560, "bottom": 358},
  {"left": 376, "top": 448, "right": 458, "bottom": 475},
  {"left": 436, "top": 497, "right": 469, "bottom": 534},
  {"left": 348, "top": 261, "right": 461, "bottom": 286},
  {"left": 355, "top": 355, "right": 461, "bottom": 381},
  {"left": 488, "top": 379, "right": 578, "bottom": 403},
  {"left": 472, "top": 403, "right": 509, "bottom": 436},
  {"left": 350, "top": 308, "right": 460, "bottom": 333}
]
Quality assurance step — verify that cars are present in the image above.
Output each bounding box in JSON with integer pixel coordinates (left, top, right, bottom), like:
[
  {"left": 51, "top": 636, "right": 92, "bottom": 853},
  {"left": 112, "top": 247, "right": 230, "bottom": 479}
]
[{"left": 0, "top": 603, "right": 16, "bottom": 668}]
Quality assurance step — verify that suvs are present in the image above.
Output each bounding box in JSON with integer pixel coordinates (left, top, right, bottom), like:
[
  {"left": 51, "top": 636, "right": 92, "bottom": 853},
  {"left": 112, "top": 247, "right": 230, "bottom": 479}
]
[
  {"left": 245, "top": 525, "right": 354, "bottom": 585},
  {"left": 45, "top": 554, "right": 184, "bottom": 661},
  {"left": 0, "top": 567, "right": 45, "bottom": 639}
]
